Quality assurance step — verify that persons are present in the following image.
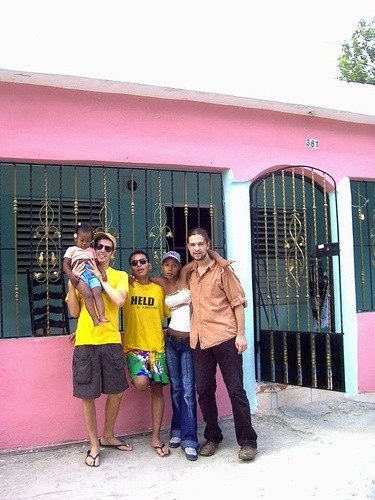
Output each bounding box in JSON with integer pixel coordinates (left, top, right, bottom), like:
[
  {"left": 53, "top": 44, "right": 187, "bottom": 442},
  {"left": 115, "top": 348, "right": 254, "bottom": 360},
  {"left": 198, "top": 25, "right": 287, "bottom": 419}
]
[
  {"left": 64, "top": 224, "right": 110, "bottom": 326},
  {"left": 128, "top": 251, "right": 236, "bottom": 461},
  {"left": 63, "top": 232, "right": 129, "bottom": 467},
  {"left": 185, "top": 227, "right": 258, "bottom": 460},
  {"left": 68, "top": 250, "right": 171, "bottom": 457}
]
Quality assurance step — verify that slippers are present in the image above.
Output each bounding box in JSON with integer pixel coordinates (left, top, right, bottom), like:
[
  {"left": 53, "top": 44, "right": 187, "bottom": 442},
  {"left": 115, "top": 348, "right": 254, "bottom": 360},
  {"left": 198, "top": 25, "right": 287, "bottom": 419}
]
[
  {"left": 98, "top": 439, "right": 133, "bottom": 451},
  {"left": 154, "top": 443, "right": 170, "bottom": 458},
  {"left": 85, "top": 450, "right": 100, "bottom": 467}
]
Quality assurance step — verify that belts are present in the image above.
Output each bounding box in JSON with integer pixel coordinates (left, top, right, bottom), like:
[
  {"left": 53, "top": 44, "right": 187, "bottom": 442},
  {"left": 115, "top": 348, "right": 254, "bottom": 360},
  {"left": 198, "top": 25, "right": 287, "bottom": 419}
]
[{"left": 167, "top": 331, "right": 189, "bottom": 341}]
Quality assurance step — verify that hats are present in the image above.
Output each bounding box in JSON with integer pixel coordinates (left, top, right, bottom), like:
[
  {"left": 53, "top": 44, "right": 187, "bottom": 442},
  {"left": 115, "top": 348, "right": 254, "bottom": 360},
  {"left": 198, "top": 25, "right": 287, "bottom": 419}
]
[
  {"left": 162, "top": 251, "right": 181, "bottom": 265},
  {"left": 94, "top": 231, "right": 116, "bottom": 259}
]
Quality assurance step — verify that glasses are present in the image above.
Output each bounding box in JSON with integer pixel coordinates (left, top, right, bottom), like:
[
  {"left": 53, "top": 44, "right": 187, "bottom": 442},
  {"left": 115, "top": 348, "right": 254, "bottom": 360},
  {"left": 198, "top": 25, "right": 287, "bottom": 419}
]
[
  {"left": 130, "top": 258, "right": 148, "bottom": 266},
  {"left": 96, "top": 243, "right": 113, "bottom": 253}
]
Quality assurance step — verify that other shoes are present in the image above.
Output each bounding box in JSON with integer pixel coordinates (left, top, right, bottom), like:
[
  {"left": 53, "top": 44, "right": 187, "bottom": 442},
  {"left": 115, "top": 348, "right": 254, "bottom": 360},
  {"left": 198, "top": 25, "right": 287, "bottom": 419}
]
[
  {"left": 238, "top": 445, "right": 257, "bottom": 459},
  {"left": 199, "top": 441, "right": 219, "bottom": 456},
  {"left": 169, "top": 436, "right": 182, "bottom": 448},
  {"left": 184, "top": 447, "right": 198, "bottom": 461}
]
